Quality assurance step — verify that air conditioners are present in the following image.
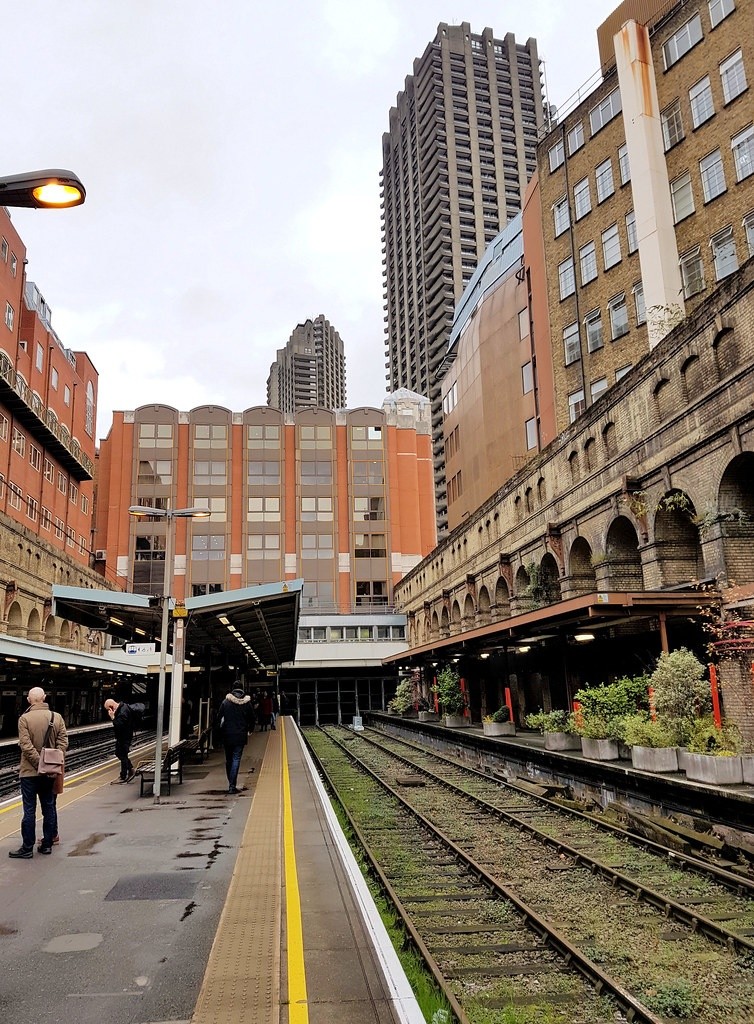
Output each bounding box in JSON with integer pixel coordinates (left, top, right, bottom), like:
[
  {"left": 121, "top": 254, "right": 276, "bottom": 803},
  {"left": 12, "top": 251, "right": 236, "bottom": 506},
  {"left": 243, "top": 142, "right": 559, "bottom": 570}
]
[{"left": 95, "top": 548, "right": 106, "bottom": 562}]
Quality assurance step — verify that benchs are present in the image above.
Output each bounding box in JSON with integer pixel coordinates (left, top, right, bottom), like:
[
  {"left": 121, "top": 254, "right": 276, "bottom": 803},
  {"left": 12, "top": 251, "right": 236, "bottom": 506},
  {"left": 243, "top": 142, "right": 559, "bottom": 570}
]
[
  {"left": 134, "top": 736, "right": 191, "bottom": 799},
  {"left": 185, "top": 724, "right": 216, "bottom": 765}
]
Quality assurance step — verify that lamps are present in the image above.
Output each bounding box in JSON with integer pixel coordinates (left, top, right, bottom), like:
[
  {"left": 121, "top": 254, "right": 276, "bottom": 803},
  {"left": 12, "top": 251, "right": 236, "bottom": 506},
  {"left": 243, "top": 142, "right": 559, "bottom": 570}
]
[
  {"left": 169, "top": 642, "right": 173, "bottom": 647},
  {"left": 245, "top": 645, "right": 251, "bottom": 650},
  {"left": 226, "top": 623, "right": 237, "bottom": 632},
  {"left": 237, "top": 637, "right": 245, "bottom": 643},
  {"left": 108, "top": 615, "right": 125, "bottom": 626},
  {"left": 432, "top": 662, "right": 438, "bottom": 667},
  {"left": 517, "top": 645, "right": 532, "bottom": 654},
  {"left": 572, "top": 634, "right": 598, "bottom": 644},
  {"left": 251, "top": 652, "right": 256, "bottom": 656},
  {"left": 453, "top": 659, "right": 459, "bottom": 663},
  {"left": 217, "top": 614, "right": 231, "bottom": 625},
  {"left": 255, "top": 657, "right": 259, "bottom": 660},
  {"left": 189, "top": 651, "right": 196, "bottom": 657},
  {"left": 479, "top": 652, "right": 490, "bottom": 660},
  {"left": 253, "top": 655, "right": 257, "bottom": 658},
  {"left": 241, "top": 641, "right": 248, "bottom": 647},
  {"left": 248, "top": 649, "right": 254, "bottom": 653},
  {"left": 153, "top": 635, "right": 161, "bottom": 642},
  {"left": 256, "top": 659, "right": 260, "bottom": 662},
  {"left": 134, "top": 627, "right": 146, "bottom": 636},
  {"left": 233, "top": 630, "right": 242, "bottom": 638}
]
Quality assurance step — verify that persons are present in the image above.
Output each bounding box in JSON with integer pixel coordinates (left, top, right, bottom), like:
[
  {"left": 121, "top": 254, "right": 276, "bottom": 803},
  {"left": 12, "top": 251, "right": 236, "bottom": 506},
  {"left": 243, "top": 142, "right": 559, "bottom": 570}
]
[
  {"left": 251, "top": 687, "right": 287, "bottom": 733},
  {"left": 104, "top": 698, "right": 137, "bottom": 785},
  {"left": 7, "top": 687, "right": 70, "bottom": 858},
  {"left": 215, "top": 682, "right": 256, "bottom": 795}
]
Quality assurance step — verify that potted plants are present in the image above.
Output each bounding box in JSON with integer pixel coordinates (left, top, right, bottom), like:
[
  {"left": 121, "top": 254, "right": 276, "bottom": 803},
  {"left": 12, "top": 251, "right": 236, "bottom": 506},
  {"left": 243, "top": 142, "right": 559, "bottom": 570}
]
[
  {"left": 561, "top": 705, "right": 620, "bottom": 762},
  {"left": 387, "top": 699, "right": 401, "bottom": 716},
  {"left": 391, "top": 695, "right": 418, "bottom": 720},
  {"left": 521, "top": 705, "right": 581, "bottom": 753},
  {"left": 681, "top": 722, "right": 743, "bottom": 786},
  {"left": 642, "top": 644, "right": 714, "bottom": 772},
  {"left": 428, "top": 663, "right": 469, "bottom": 728},
  {"left": 615, "top": 709, "right": 679, "bottom": 774},
  {"left": 480, "top": 705, "right": 517, "bottom": 739},
  {"left": 386, "top": 675, "right": 440, "bottom": 725}
]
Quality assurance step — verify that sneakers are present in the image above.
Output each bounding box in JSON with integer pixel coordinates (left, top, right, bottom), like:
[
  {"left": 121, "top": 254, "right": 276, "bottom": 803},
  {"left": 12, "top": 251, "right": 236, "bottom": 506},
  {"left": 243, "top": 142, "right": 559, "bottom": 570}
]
[
  {"left": 37, "top": 846, "right": 51, "bottom": 854},
  {"left": 111, "top": 777, "right": 126, "bottom": 784},
  {"left": 127, "top": 769, "right": 136, "bottom": 782},
  {"left": 229, "top": 788, "right": 242, "bottom": 793},
  {"left": 9, "top": 848, "right": 33, "bottom": 858}
]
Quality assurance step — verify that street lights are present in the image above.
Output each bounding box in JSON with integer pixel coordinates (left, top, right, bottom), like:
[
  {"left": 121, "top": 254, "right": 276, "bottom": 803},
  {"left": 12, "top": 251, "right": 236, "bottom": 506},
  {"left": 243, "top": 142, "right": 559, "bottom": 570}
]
[{"left": 128, "top": 506, "right": 211, "bottom": 804}]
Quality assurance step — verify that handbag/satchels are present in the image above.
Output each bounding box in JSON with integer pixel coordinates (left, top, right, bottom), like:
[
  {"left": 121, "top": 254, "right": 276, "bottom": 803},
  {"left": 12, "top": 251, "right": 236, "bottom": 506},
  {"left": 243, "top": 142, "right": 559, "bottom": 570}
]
[{"left": 37, "top": 746, "right": 65, "bottom": 775}]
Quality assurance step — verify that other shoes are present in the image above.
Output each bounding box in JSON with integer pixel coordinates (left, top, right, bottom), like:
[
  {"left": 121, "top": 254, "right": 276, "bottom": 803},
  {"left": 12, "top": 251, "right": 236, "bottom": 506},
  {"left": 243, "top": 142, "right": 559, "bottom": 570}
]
[{"left": 38, "top": 835, "right": 59, "bottom": 844}]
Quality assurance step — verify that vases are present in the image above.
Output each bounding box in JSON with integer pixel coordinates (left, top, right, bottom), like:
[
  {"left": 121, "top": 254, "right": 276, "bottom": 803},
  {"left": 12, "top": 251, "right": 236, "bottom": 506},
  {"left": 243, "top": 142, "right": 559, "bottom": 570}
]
[{"left": 741, "top": 753, "right": 754, "bottom": 784}]
